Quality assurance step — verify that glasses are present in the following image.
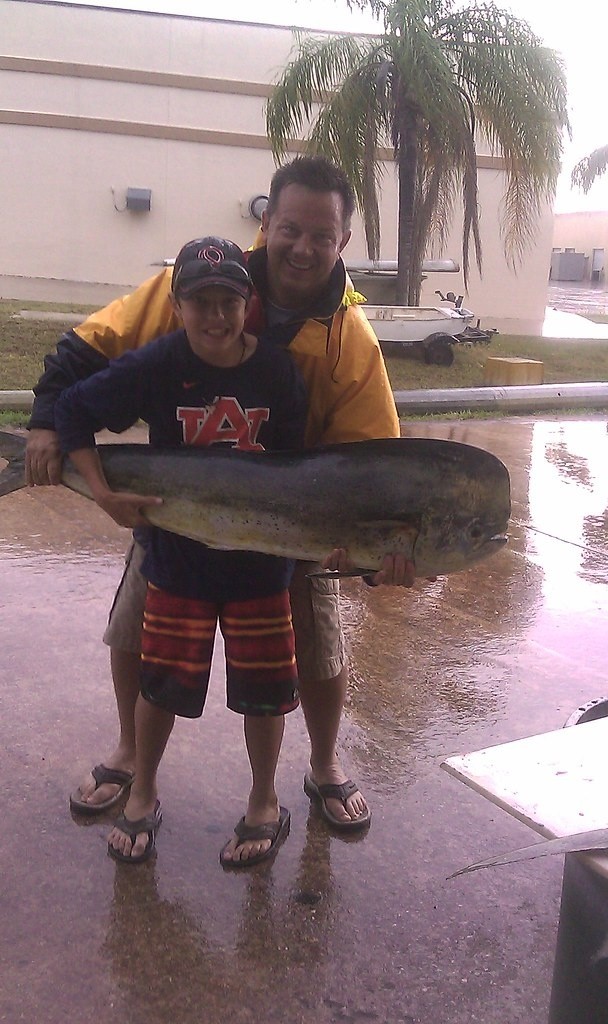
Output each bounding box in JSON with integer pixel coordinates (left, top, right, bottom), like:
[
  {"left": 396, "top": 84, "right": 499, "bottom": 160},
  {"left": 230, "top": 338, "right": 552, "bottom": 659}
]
[{"left": 170, "top": 259, "right": 254, "bottom": 299}]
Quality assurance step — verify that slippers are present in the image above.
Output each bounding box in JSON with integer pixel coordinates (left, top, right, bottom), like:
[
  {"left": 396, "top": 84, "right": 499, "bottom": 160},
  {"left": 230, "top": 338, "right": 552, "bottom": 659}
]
[
  {"left": 106, "top": 798, "right": 164, "bottom": 866},
  {"left": 217, "top": 804, "right": 292, "bottom": 869},
  {"left": 68, "top": 761, "right": 137, "bottom": 814},
  {"left": 305, "top": 773, "right": 372, "bottom": 832}
]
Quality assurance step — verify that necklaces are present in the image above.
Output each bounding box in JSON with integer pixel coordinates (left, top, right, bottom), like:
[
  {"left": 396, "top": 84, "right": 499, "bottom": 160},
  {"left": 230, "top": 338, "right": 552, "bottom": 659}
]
[{"left": 237, "top": 328, "right": 247, "bottom": 367}]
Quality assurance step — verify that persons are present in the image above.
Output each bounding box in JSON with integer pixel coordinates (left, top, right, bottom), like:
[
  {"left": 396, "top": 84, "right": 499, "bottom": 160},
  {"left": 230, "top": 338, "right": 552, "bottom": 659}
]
[
  {"left": 24, "top": 158, "right": 436, "bottom": 834},
  {"left": 53, "top": 237, "right": 356, "bottom": 866}
]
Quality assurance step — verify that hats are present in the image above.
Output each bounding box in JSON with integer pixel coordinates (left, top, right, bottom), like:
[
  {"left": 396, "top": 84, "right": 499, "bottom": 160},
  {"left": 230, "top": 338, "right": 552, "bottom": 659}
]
[{"left": 171, "top": 237, "right": 251, "bottom": 303}]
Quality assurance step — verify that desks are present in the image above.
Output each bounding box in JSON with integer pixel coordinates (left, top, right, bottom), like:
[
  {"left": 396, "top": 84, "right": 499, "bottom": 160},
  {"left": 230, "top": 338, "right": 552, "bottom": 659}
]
[{"left": 438, "top": 717, "right": 607, "bottom": 1024}]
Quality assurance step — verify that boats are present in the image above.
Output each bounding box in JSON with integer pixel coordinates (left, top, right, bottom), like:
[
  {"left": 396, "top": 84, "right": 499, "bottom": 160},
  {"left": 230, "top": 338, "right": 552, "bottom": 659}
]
[{"left": 358, "top": 303, "right": 474, "bottom": 342}]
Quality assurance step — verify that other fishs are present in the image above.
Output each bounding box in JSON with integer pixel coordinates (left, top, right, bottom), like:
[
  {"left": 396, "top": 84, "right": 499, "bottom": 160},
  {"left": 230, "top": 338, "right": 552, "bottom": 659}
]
[{"left": 0, "top": 428, "right": 512, "bottom": 590}]
